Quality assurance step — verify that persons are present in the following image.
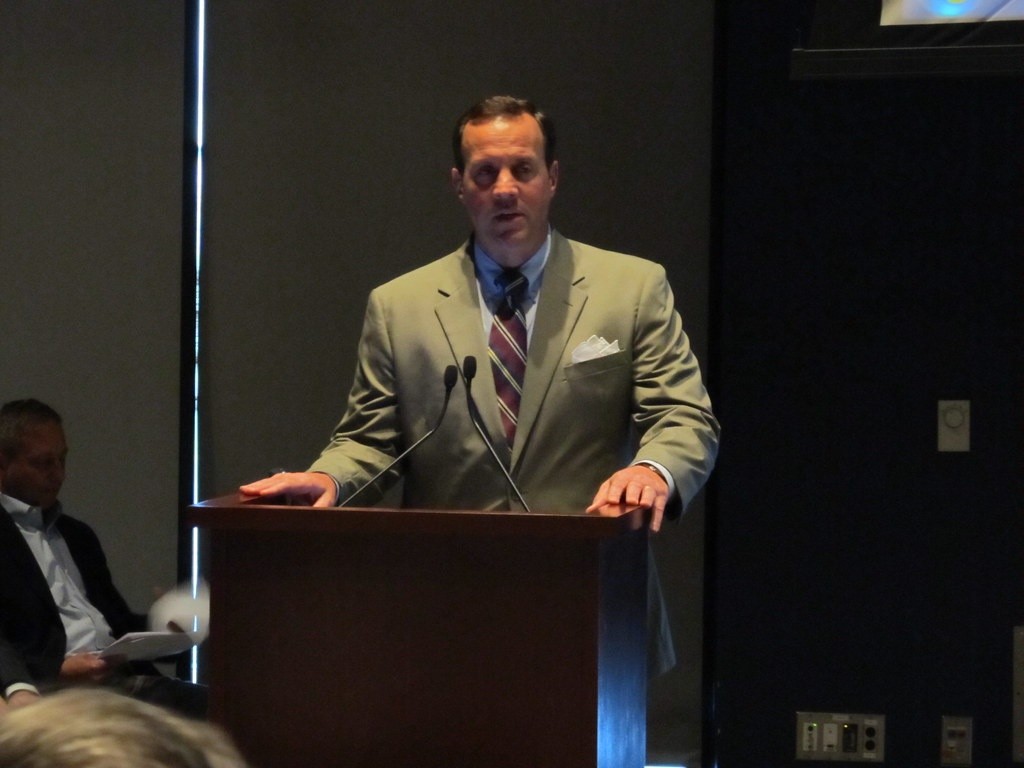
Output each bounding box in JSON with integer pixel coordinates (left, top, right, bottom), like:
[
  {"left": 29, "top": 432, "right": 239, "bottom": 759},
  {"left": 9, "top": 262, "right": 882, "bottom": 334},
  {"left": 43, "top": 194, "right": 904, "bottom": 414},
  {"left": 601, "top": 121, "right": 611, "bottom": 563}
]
[
  {"left": 0, "top": 398, "right": 211, "bottom": 726},
  {"left": 0, "top": 625, "right": 41, "bottom": 714},
  {"left": 0, "top": 688, "right": 248, "bottom": 768},
  {"left": 240, "top": 95, "right": 720, "bottom": 687}
]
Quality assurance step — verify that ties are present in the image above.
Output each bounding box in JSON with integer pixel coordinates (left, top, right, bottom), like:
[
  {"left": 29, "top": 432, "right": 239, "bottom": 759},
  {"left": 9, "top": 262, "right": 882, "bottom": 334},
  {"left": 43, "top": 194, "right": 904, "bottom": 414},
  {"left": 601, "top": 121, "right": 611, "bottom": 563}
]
[{"left": 488, "top": 269, "right": 533, "bottom": 451}]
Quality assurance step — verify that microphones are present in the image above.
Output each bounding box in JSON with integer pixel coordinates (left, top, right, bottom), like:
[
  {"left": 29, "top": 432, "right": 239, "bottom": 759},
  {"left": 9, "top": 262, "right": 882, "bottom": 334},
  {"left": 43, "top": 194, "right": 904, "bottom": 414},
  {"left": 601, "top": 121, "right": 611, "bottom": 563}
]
[
  {"left": 463, "top": 356, "right": 529, "bottom": 513},
  {"left": 339, "top": 364, "right": 458, "bottom": 507}
]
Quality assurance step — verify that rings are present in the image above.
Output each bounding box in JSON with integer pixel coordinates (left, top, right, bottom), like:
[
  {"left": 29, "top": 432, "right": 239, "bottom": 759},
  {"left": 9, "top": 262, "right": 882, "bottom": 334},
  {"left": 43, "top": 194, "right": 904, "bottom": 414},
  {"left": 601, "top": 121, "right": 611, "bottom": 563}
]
[{"left": 282, "top": 471, "right": 285, "bottom": 474}]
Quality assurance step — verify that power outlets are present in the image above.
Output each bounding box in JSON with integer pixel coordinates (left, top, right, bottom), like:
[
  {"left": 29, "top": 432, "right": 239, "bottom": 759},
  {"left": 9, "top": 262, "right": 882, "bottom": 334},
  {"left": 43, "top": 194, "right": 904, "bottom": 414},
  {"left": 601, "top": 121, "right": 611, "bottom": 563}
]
[
  {"left": 941, "top": 715, "right": 973, "bottom": 766},
  {"left": 796, "top": 711, "right": 885, "bottom": 763}
]
